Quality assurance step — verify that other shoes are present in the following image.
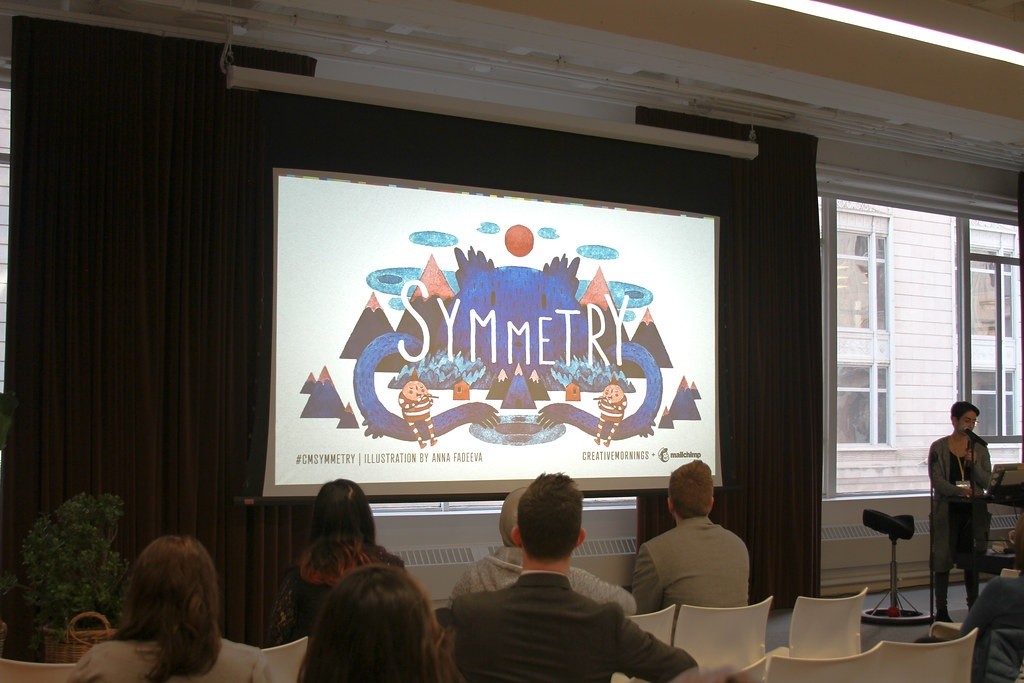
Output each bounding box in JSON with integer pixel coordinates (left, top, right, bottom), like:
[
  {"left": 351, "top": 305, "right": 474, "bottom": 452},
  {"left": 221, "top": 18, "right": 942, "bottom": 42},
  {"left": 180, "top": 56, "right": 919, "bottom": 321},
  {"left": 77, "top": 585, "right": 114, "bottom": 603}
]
[
  {"left": 934, "top": 604, "right": 954, "bottom": 623},
  {"left": 966, "top": 596, "right": 980, "bottom": 613}
]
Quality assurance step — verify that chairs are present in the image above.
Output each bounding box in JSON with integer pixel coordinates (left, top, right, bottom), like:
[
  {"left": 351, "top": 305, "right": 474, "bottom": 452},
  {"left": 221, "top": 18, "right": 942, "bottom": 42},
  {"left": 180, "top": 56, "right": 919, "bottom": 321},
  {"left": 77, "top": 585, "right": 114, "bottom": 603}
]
[{"left": 0, "top": 587, "right": 979, "bottom": 683}]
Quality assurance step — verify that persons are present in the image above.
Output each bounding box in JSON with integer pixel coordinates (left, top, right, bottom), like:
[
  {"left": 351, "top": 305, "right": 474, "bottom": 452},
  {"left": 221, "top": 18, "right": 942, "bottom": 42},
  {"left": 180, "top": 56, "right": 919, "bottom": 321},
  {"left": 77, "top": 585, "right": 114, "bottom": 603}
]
[
  {"left": 449, "top": 471, "right": 699, "bottom": 683},
  {"left": 928, "top": 401, "right": 992, "bottom": 622},
  {"left": 634, "top": 457, "right": 750, "bottom": 618},
  {"left": 66, "top": 535, "right": 273, "bottom": 682},
  {"left": 265, "top": 478, "right": 442, "bottom": 683},
  {"left": 913, "top": 513, "right": 1024, "bottom": 682}
]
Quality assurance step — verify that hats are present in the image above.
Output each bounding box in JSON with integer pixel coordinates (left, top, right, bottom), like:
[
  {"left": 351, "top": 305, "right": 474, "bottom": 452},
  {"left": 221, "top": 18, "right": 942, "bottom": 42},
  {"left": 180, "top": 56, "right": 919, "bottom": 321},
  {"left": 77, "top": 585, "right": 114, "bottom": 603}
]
[{"left": 499, "top": 488, "right": 530, "bottom": 548}]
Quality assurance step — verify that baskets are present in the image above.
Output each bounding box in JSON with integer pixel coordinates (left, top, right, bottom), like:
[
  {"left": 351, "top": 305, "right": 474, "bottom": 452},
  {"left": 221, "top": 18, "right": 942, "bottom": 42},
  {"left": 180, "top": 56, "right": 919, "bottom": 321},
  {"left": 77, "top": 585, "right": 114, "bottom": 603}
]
[{"left": 42, "top": 611, "right": 117, "bottom": 663}]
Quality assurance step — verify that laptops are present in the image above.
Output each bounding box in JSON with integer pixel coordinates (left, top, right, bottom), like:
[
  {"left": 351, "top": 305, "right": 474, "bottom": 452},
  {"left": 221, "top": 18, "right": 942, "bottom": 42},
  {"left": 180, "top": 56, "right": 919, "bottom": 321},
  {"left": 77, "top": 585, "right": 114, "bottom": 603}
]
[{"left": 974, "top": 463, "right": 1023, "bottom": 495}]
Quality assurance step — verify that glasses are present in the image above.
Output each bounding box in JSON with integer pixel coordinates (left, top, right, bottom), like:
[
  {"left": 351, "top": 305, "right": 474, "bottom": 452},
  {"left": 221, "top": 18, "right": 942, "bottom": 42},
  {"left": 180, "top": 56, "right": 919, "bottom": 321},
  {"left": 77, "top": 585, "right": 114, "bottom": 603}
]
[{"left": 958, "top": 416, "right": 979, "bottom": 424}]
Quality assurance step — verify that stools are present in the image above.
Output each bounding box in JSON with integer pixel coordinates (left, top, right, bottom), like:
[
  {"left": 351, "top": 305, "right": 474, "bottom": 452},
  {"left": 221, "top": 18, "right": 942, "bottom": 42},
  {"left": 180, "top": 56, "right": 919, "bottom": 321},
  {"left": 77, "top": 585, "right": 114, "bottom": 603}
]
[{"left": 858, "top": 509, "right": 934, "bottom": 625}]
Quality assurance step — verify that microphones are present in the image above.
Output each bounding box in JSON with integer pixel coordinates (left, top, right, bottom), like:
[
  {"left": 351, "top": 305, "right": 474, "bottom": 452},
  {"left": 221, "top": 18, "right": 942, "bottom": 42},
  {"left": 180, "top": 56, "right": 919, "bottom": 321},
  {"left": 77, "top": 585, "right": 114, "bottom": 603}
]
[
  {"left": 965, "top": 439, "right": 972, "bottom": 468},
  {"left": 964, "top": 426, "right": 988, "bottom": 447}
]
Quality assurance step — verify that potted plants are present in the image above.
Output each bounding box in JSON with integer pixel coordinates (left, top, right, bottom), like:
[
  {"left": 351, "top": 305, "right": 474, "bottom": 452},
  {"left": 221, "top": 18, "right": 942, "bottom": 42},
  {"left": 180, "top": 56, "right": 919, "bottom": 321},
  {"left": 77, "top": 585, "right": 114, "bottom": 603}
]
[{"left": 20, "top": 492, "right": 137, "bottom": 663}]
